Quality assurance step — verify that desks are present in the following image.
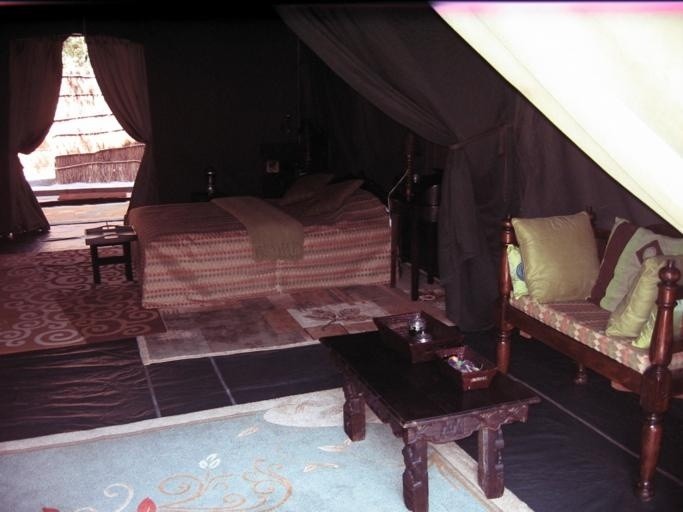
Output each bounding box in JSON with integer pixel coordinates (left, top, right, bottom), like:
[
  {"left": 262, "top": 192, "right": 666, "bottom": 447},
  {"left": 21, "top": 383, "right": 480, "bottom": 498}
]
[{"left": 388, "top": 166, "right": 440, "bottom": 301}]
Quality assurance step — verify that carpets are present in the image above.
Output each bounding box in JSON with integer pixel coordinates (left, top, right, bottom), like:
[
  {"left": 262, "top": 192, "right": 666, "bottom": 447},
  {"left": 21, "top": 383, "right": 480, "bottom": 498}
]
[
  {"left": 1, "top": 386, "right": 535, "bottom": 512},
  {"left": 0, "top": 247, "right": 448, "bottom": 367}
]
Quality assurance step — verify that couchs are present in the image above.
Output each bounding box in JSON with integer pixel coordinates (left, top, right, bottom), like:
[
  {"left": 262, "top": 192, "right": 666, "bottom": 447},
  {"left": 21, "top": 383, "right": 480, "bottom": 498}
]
[{"left": 495, "top": 207, "right": 683, "bottom": 502}]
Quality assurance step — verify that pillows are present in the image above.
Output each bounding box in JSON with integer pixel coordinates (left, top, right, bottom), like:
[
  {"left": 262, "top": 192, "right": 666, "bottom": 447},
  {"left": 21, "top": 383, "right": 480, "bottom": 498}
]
[
  {"left": 505, "top": 211, "right": 683, "bottom": 349},
  {"left": 278, "top": 170, "right": 389, "bottom": 223}
]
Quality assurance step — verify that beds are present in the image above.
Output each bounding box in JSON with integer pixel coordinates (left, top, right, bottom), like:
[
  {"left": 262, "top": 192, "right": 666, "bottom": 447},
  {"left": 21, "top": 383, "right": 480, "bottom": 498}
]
[{"left": 128, "top": 173, "right": 392, "bottom": 314}]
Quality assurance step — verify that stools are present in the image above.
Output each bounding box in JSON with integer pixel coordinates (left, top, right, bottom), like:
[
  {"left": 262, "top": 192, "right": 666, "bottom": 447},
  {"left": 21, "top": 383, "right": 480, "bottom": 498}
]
[{"left": 84, "top": 224, "right": 136, "bottom": 284}]
